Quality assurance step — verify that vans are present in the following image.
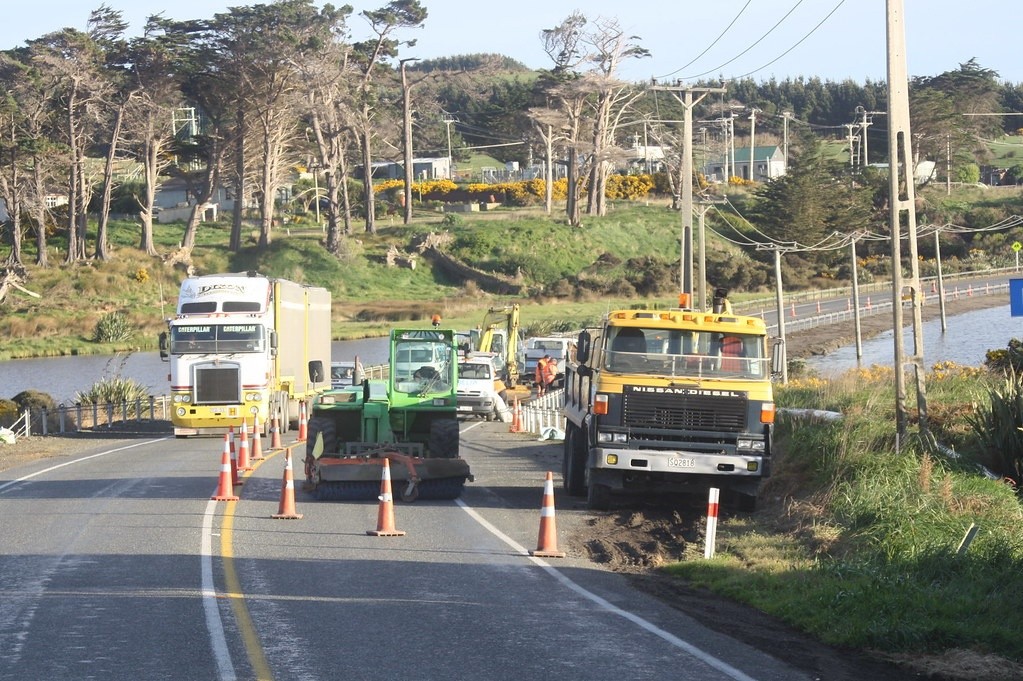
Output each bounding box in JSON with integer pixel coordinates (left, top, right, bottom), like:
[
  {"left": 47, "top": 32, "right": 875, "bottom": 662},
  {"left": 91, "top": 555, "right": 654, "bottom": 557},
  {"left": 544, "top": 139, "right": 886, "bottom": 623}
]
[
  {"left": 456, "top": 355, "right": 495, "bottom": 422},
  {"left": 388, "top": 344, "right": 446, "bottom": 393}
]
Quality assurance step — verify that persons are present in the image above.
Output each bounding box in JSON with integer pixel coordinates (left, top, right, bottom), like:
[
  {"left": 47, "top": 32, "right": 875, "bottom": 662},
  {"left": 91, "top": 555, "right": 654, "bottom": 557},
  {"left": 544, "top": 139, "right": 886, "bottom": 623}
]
[
  {"left": 536, "top": 355, "right": 564, "bottom": 396},
  {"left": 345, "top": 369, "right": 353, "bottom": 379}
]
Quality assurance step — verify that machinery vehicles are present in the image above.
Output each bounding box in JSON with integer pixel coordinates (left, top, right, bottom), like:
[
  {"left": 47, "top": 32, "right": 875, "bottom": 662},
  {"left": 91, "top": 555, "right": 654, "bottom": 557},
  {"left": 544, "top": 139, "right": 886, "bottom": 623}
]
[
  {"left": 477, "top": 303, "right": 532, "bottom": 403},
  {"left": 302, "top": 314, "right": 475, "bottom": 501}
]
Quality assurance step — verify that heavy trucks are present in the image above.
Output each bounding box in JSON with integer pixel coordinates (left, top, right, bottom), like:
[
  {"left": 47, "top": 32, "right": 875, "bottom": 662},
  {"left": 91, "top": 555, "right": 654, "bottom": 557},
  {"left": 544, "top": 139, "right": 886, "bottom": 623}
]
[{"left": 157, "top": 269, "right": 333, "bottom": 439}]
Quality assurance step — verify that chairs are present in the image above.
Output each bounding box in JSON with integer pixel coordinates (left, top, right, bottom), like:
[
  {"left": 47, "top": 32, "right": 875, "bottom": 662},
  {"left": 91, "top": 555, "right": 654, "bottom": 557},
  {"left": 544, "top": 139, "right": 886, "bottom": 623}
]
[
  {"left": 413, "top": 367, "right": 441, "bottom": 391},
  {"left": 715, "top": 336, "right": 749, "bottom": 373},
  {"left": 611, "top": 328, "right": 649, "bottom": 368}
]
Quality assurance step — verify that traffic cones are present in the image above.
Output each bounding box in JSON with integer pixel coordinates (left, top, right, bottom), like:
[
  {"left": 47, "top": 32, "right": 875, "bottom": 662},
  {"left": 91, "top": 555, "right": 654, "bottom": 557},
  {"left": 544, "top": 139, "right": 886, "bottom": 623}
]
[
  {"left": 267, "top": 409, "right": 284, "bottom": 450},
  {"left": 296, "top": 402, "right": 307, "bottom": 441},
  {"left": 250, "top": 412, "right": 266, "bottom": 461},
  {"left": 510, "top": 395, "right": 527, "bottom": 433},
  {"left": 211, "top": 434, "right": 239, "bottom": 501},
  {"left": 270, "top": 447, "right": 304, "bottom": 520},
  {"left": 528, "top": 471, "right": 566, "bottom": 558},
  {"left": 791, "top": 302, "right": 796, "bottom": 316},
  {"left": 368, "top": 458, "right": 406, "bottom": 537},
  {"left": 236, "top": 416, "right": 253, "bottom": 471},
  {"left": 816, "top": 300, "right": 821, "bottom": 313},
  {"left": 760, "top": 309, "right": 764, "bottom": 320},
  {"left": 229, "top": 425, "right": 244, "bottom": 485}
]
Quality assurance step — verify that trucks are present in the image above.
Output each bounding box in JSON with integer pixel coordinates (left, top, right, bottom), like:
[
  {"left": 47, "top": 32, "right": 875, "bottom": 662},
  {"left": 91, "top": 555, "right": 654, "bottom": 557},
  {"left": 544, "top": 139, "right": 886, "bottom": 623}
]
[
  {"left": 560, "top": 287, "right": 784, "bottom": 506},
  {"left": 522, "top": 337, "right": 578, "bottom": 373}
]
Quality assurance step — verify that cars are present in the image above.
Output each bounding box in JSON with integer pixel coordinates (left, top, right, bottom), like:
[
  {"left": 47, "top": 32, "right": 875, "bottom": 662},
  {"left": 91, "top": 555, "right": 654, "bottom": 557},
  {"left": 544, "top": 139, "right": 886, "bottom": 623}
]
[
  {"left": 548, "top": 332, "right": 562, "bottom": 338},
  {"left": 331, "top": 362, "right": 365, "bottom": 390}
]
